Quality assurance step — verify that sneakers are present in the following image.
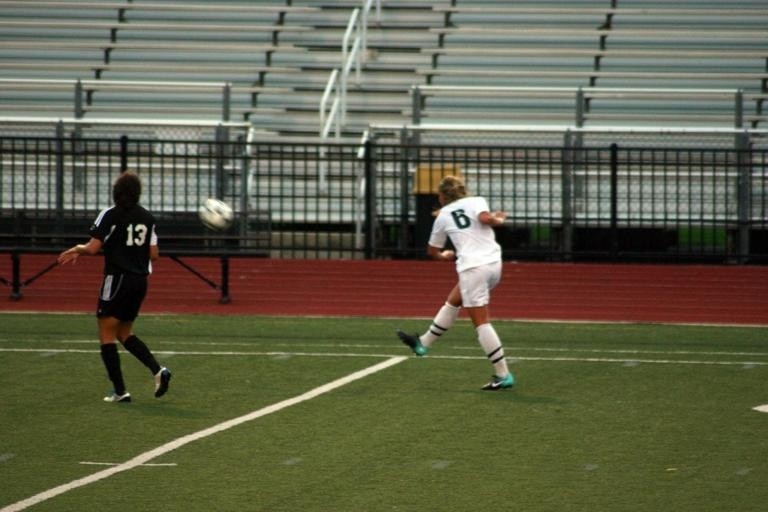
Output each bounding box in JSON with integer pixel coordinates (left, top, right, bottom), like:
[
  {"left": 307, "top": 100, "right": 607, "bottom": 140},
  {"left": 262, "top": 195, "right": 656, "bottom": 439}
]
[
  {"left": 154, "top": 368, "right": 171, "bottom": 397},
  {"left": 396, "top": 331, "right": 430, "bottom": 356},
  {"left": 481, "top": 373, "right": 514, "bottom": 391},
  {"left": 104, "top": 392, "right": 131, "bottom": 401}
]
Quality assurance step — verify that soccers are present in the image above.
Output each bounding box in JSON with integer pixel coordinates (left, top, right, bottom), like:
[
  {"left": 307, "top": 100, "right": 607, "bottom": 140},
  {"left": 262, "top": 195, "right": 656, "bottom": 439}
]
[{"left": 198, "top": 198, "right": 234, "bottom": 233}]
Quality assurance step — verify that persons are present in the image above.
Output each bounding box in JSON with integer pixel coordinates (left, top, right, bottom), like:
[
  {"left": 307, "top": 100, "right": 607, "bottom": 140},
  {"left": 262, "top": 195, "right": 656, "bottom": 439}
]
[
  {"left": 57, "top": 169, "right": 172, "bottom": 403},
  {"left": 395, "top": 173, "right": 516, "bottom": 390}
]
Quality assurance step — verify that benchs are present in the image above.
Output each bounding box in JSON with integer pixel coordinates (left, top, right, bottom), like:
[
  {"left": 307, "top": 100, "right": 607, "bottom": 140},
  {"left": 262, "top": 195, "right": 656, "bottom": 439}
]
[{"left": 0, "top": 0, "right": 768, "bottom": 229}]
